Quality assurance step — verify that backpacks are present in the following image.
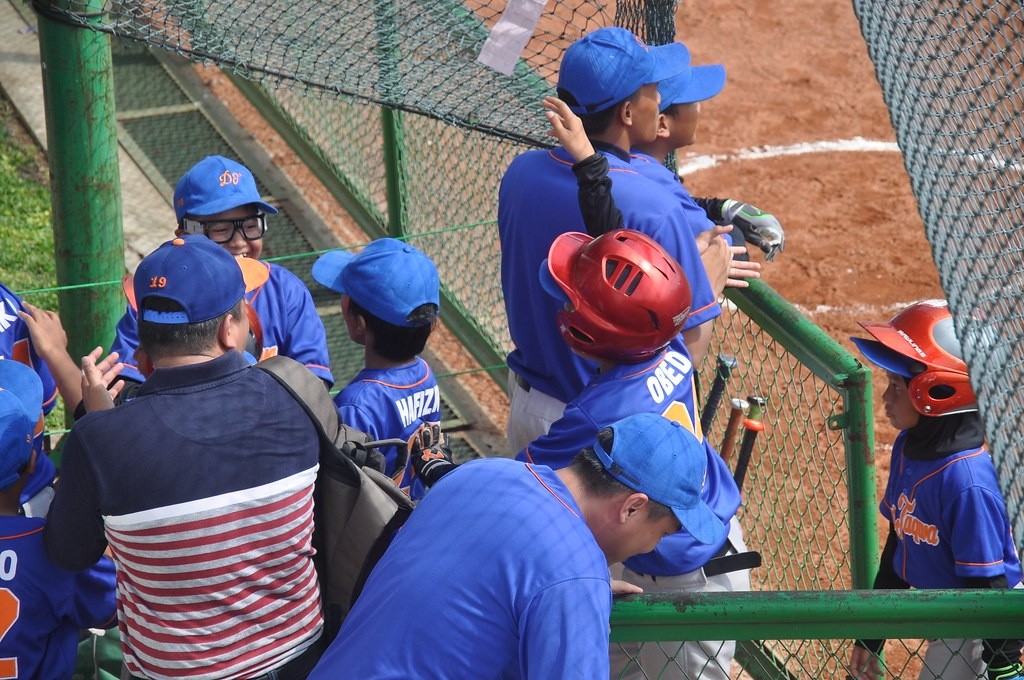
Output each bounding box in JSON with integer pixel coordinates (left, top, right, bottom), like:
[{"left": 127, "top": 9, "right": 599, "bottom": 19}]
[{"left": 252, "top": 354, "right": 415, "bottom": 640}]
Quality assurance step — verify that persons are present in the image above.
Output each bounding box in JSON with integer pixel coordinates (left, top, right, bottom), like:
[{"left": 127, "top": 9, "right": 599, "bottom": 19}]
[
  {"left": 499, "top": 28, "right": 762, "bottom": 456},
  {"left": 850, "top": 300, "right": 1024, "bottom": 680},
  {"left": 17, "top": 232, "right": 326, "bottom": 680},
  {"left": 413, "top": 97, "right": 763, "bottom": 679},
  {"left": 111, "top": 155, "right": 336, "bottom": 406},
  {"left": 305, "top": 413, "right": 724, "bottom": 680},
  {"left": 0, "top": 285, "right": 117, "bottom": 680},
  {"left": 311, "top": 237, "right": 444, "bottom": 503}
]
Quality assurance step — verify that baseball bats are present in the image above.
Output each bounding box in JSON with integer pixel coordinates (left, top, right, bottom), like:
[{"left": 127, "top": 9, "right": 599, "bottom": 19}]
[
  {"left": 720, "top": 398, "right": 750, "bottom": 466},
  {"left": 701, "top": 354, "right": 738, "bottom": 438},
  {"left": 733, "top": 396, "right": 768, "bottom": 494}
]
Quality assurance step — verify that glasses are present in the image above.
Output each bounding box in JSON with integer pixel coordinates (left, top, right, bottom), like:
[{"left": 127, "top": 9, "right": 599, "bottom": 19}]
[{"left": 178, "top": 213, "right": 265, "bottom": 244}]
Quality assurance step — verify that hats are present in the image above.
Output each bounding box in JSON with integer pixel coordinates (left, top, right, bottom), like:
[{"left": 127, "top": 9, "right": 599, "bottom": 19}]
[
  {"left": 593, "top": 412, "right": 725, "bottom": 545},
  {"left": 134, "top": 233, "right": 269, "bottom": 323},
  {"left": 0, "top": 359, "right": 43, "bottom": 491},
  {"left": 850, "top": 337, "right": 953, "bottom": 398},
  {"left": 174, "top": 157, "right": 278, "bottom": 223},
  {"left": 539, "top": 258, "right": 657, "bottom": 324},
  {"left": 657, "top": 65, "right": 726, "bottom": 114},
  {"left": 557, "top": 27, "right": 690, "bottom": 114},
  {"left": 311, "top": 238, "right": 440, "bottom": 328}
]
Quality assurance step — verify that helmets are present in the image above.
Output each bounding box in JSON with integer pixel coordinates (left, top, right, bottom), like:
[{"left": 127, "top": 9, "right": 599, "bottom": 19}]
[
  {"left": 856, "top": 298, "right": 1006, "bottom": 417},
  {"left": 548, "top": 228, "right": 691, "bottom": 364}
]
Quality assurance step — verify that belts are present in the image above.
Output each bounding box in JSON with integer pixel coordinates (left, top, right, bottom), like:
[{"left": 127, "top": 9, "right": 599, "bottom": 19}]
[
  {"left": 632, "top": 539, "right": 761, "bottom": 581},
  {"left": 255, "top": 633, "right": 329, "bottom": 680},
  {"left": 516, "top": 373, "right": 531, "bottom": 393}
]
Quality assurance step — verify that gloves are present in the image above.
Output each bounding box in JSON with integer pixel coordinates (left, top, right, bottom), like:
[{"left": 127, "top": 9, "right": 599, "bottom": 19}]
[
  {"left": 411, "top": 425, "right": 460, "bottom": 489},
  {"left": 720, "top": 199, "right": 785, "bottom": 262}
]
